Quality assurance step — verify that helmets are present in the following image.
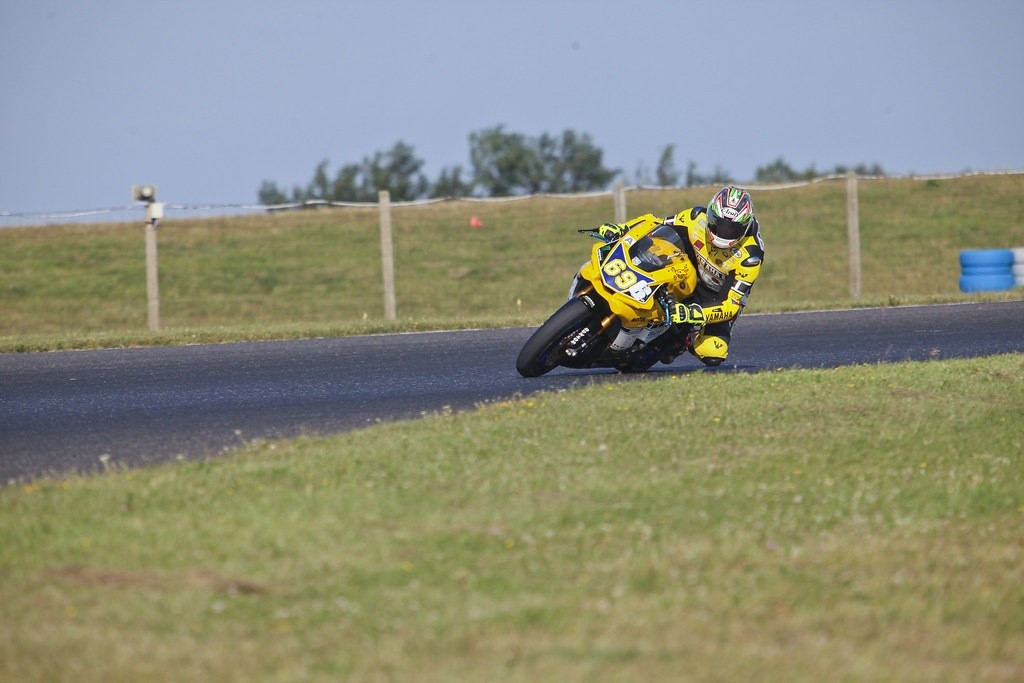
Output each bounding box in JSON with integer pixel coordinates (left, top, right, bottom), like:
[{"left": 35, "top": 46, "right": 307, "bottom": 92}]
[{"left": 705, "top": 186, "right": 755, "bottom": 249}]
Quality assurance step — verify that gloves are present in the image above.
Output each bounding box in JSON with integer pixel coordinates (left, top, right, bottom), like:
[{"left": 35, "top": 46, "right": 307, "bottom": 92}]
[
  {"left": 598, "top": 222, "right": 630, "bottom": 242},
  {"left": 668, "top": 300, "right": 707, "bottom": 328}
]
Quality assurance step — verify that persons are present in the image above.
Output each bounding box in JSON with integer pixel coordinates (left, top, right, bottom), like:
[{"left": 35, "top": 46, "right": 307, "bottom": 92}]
[{"left": 600, "top": 185, "right": 765, "bottom": 366}]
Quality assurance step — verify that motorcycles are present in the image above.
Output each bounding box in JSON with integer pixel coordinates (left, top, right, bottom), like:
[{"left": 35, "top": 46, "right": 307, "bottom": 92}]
[{"left": 516, "top": 212, "right": 703, "bottom": 379}]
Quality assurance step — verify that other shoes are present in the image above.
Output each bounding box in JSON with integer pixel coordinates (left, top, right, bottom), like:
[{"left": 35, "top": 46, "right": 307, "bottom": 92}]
[{"left": 660, "top": 329, "right": 698, "bottom": 364}]
